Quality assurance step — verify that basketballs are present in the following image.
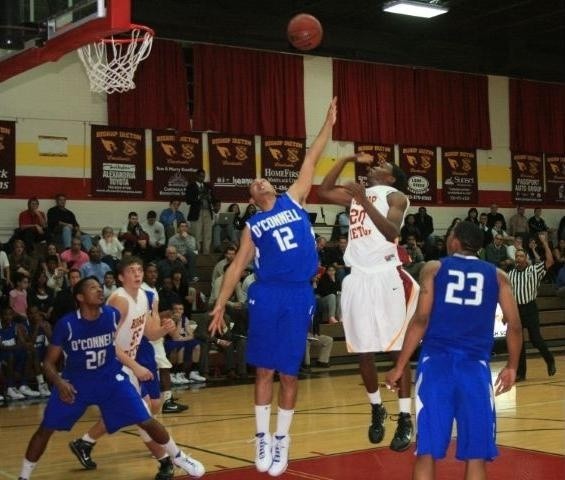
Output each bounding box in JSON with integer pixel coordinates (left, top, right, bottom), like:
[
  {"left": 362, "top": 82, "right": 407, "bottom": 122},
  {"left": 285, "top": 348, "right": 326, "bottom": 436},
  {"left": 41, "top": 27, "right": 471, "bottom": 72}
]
[{"left": 287, "top": 13, "right": 323, "bottom": 51}]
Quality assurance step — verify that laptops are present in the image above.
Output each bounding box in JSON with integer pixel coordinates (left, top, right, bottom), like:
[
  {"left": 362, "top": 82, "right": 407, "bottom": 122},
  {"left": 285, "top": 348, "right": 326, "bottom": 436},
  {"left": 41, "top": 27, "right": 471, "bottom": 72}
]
[{"left": 217, "top": 212, "right": 235, "bottom": 225}]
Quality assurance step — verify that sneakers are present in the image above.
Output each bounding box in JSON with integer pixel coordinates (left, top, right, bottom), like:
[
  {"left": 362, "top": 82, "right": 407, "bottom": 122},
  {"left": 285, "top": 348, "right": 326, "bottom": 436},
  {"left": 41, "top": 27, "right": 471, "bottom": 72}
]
[
  {"left": 368, "top": 403, "right": 387, "bottom": 443},
  {"left": 68, "top": 438, "right": 97, "bottom": 469},
  {"left": 172, "top": 452, "right": 205, "bottom": 477},
  {"left": 390, "top": 412, "right": 414, "bottom": 452},
  {"left": 189, "top": 371, "right": 206, "bottom": 381},
  {"left": 516, "top": 375, "right": 526, "bottom": 381},
  {"left": 20, "top": 385, "right": 40, "bottom": 398},
  {"left": 7, "top": 387, "right": 25, "bottom": 400},
  {"left": 162, "top": 402, "right": 189, "bottom": 412},
  {"left": 330, "top": 317, "right": 338, "bottom": 323},
  {"left": 268, "top": 432, "right": 291, "bottom": 477},
  {"left": 547, "top": 361, "right": 556, "bottom": 376},
  {"left": 169, "top": 374, "right": 177, "bottom": 384},
  {"left": 255, "top": 432, "right": 272, "bottom": 472},
  {"left": 155, "top": 457, "right": 175, "bottom": 480},
  {"left": 39, "top": 384, "right": 52, "bottom": 398},
  {"left": 177, "top": 373, "right": 190, "bottom": 383}
]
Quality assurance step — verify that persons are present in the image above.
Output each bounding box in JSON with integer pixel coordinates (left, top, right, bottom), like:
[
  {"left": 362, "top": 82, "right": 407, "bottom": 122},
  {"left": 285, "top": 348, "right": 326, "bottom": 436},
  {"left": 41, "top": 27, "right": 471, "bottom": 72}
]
[
  {"left": 47, "top": 193, "right": 94, "bottom": 250},
  {"left": 509, "top": 205, "right": 528, "bottom": 236},
  {"left": 211, "top": 199, "right": 240, "bottom": 251},
  {"left": 463, "top": 207, "right": 479, "bottom": 225},
  {"left": 207, "top": 264, "right": 248, "bottom": 312},
  {"left": 241, "top": 203, "right": 257, "bottom": 231},
  {"left": 117, "top": 211, "right": 150, "bottom": 250},
  {"left": 552, "top": 239, "right": 565, "bottom": 288},
  {"left": 402, "top": 235, "right": 426, "bottom": 276},
  {"left": 528, "top": 206, "right": 555, "bottom": 233},
  {"left": 502, "top": 231, "right": 557, "bottom": 382},
  {"left": 171, "top": 268, "right": 193, "bottom": 311},
  {"left": 383, "top": 220, "right": 524, "bottom": 480},
  {"left": 317, "top": 264, "right": 342, "bottom": 325},
  {"left": 97, "top": 226, "right": 124, "bottom": 269},
  {"left": 210, "top": 247, "right": 251, "bottom": 285},
  {"left": 479, "top": 233, "right": 514, "bottom": 272},
  {"left": 443, "top": 217, "right": 461, "bottom": 246},
  {"left": 331, "top": 236, "right": 350, "bottom": 282},
  {"left": 317, "top": 152, "right": 420, "bottom": 453},
  {"left": 135, "top": 290, "right": 162, "bottom": 415},
  {"left": 60, "top": 237, "right": 91, "bottom": 272},
  {"left": 132, "top": 233, "right": 158, "bottom": 264},
  {"left": 81, "top": 246, "right": 112, "bottom": 284},
  {"left": 487, "top": 203, "right": 507, "bottom": 231},
  {"left": 17, "top": 276, "right": 207, "bottom": 480},
  {"left": 56, "top": 268, "right": 83, "bottom": 319},
  {"left": 164, "top": 341, "right": 183, "bottom": 385},
  {"left": 197, "top": 298, "right": 242, "bottom": 381},
  {"left": 0, "top": 237, "right": 59, "bottom": 402},
  {"left": 141, "top": 260, "right": 190, "bottom": 414},
  {"left": 228, "top": 203, "right": 242, "bottom": 229},
  {"left": 18, "top": 196, "right": 47, "bottom": 235},
  {"left": 316, "top": 236, "right": 327, "bottom": 266},
  {"left": 166, "top": 219, "right": 201, "bottom": 283},
  {"left": 101, "top": 270, "right": 120, "bottom": 300},
  {"left": 207, "top": 96, "right": 337, "bottom": 477},
  {"left": 164, "top": 301, "right": 207, "bottom": 384},
  {"left": 155, "top": 245, "right": 187, "bottom": 291},
  {"left": 68, "top": 256, "right": 176, "bottom": 480},
  {"left": 415, "top": 206, "right": 435, "bottom": 237},
  {"left": 479, "top": 212, "right": 489, "bottom": 235},
  {"left": 140, "top": 210, "right": 166, "bottom": 248},
  {"left": 506, "top": 235, "right": 533, "bottom": 267},
  {"left": 158, "top": 196, "right": 187, "bottom": 242},
  {"left": 491, "top": 220, "right": 515, "bottom": 241},
  {"left": 399, "top": 214, "right": 425, "bottom": 246},
  {"left": 184, "top": 167, "right": 216, "bottom": 257}
]
